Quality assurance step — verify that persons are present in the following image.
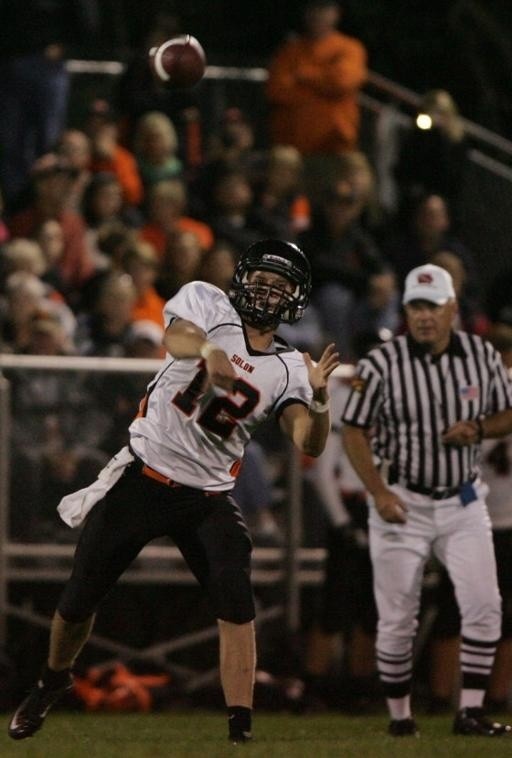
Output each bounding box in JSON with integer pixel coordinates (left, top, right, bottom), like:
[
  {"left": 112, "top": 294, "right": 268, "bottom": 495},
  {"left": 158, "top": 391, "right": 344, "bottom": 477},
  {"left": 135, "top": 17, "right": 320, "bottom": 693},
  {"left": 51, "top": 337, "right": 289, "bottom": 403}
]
[
  {"left": 8, "top": 238, "right": 341, "bottom": 741},
  {"left": 341, "top": 264, "right": 512, "bottom": 737},
  {"left": 1, "top": 1, "right": 512, "bottom": 550}
]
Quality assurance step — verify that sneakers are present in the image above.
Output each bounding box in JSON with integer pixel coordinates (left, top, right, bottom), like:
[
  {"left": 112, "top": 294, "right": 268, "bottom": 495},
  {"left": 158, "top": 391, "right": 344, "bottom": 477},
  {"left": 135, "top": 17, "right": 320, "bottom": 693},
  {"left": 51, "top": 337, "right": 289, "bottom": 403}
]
[
  {"left": 453, "top": 707, "right": 512, "bottom": 735},
  {"left": 9, "top": 672, "right": 75, "bottom": 741}
]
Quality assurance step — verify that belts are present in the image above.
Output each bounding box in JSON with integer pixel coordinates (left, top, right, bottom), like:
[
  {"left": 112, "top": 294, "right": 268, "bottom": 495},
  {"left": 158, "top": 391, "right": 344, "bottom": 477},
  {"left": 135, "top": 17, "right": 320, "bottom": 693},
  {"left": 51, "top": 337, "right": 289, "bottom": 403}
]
[
  {"left": 130, "top": 458, "right": 225, "bottom": 501},
  {"left": 384, "top": 466, "right": 480, "bottom": 501}
]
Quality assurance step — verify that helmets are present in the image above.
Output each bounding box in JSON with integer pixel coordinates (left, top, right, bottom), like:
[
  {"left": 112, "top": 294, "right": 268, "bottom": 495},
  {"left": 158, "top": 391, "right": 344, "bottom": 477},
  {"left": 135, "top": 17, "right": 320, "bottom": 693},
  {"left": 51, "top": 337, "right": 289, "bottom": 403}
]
[{"left": 228, "top": 236, "right": 313, "bottom": 295}]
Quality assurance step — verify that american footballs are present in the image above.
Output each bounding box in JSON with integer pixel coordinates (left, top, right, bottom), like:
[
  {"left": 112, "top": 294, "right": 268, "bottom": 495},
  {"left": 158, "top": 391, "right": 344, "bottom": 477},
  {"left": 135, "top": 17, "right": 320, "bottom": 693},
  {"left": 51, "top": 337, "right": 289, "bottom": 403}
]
[{"left": 148, "top": 34, "right": 206, "bottom": 89}]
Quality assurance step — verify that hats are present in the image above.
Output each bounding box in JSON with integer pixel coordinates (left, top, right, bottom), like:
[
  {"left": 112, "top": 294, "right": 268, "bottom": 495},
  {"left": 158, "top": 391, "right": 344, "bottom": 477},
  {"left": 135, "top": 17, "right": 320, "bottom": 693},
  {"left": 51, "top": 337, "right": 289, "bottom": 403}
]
[{"left": 402, "top": 263, "right": 460, "bottom": 308}]
